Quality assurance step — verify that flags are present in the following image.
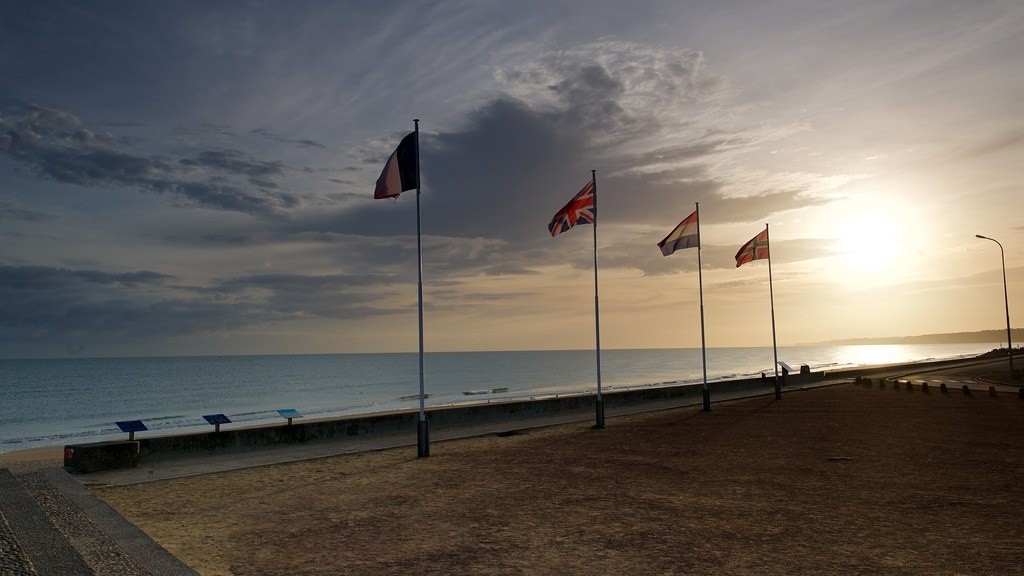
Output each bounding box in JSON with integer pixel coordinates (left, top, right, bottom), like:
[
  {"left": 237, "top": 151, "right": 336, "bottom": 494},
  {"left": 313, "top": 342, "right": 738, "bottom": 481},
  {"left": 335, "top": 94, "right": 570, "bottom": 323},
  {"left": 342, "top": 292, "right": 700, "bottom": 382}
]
[
  {"left": 735, "top": 229, "right": 769, "bottom": 268},
  {"left": 374, "top": 132, "right": 418, "bottom": 199},
  {"left": 657, "top": 211, "right": 699, "bottom": 257},
  {"left": 547, "top": 182, "right": 596, "bottom": 237}
]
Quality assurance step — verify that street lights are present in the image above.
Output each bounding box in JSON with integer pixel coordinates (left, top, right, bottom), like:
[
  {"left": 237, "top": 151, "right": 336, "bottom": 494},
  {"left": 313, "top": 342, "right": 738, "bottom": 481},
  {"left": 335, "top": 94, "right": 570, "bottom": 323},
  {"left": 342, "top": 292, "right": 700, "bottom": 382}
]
[{"left": 975, "top": 234, "right": 1012, "bottom": 370}]
